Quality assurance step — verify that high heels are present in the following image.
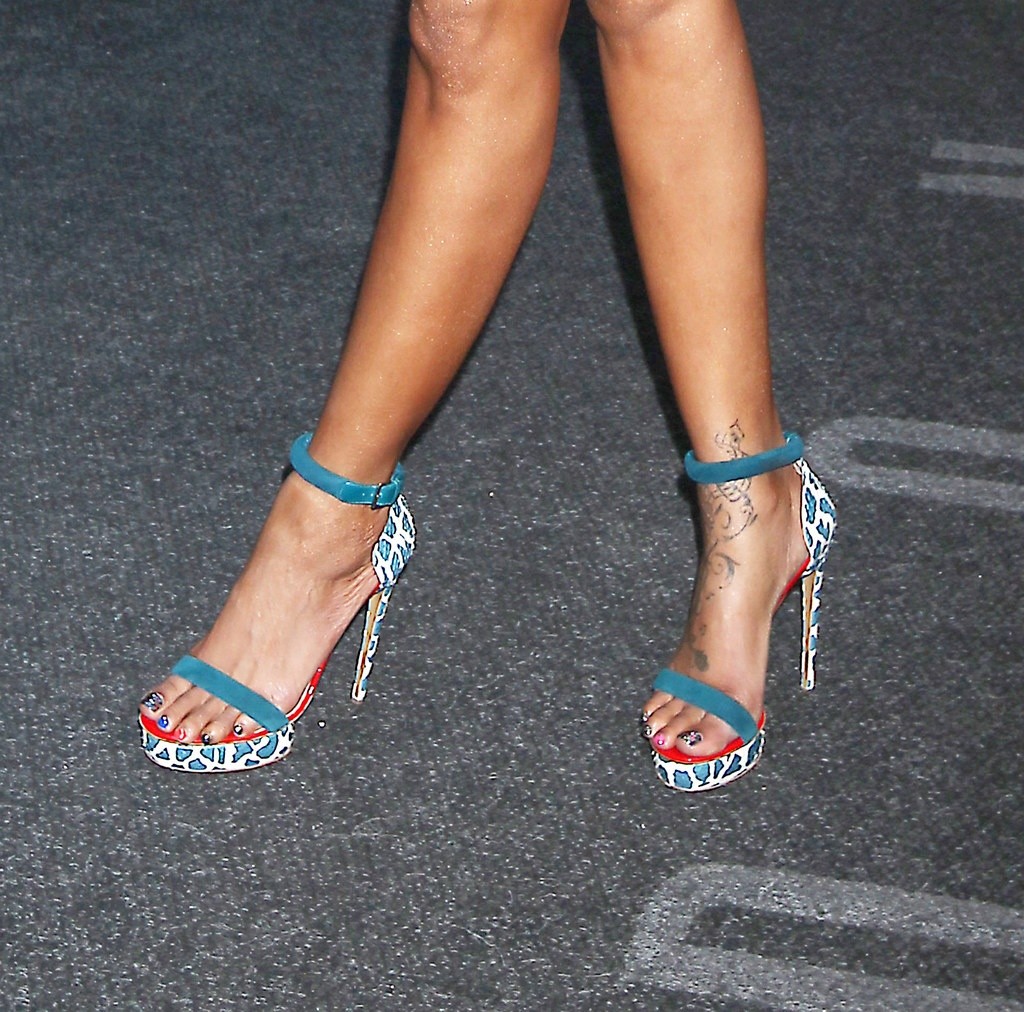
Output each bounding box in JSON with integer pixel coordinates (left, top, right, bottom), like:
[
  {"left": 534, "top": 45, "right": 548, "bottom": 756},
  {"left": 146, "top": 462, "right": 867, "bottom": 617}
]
[
  {"left": 637, "top": 430, "right": 838, "bottom": 794},
  {"left": 136, "top": 432, "right": 417, "bottom": 775}
]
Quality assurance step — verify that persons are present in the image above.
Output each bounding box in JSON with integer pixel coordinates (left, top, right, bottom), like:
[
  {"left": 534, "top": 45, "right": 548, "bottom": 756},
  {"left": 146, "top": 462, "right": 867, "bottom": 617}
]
[{"left": 139, "top": 0, "right": 841, "bottom": 795}]
